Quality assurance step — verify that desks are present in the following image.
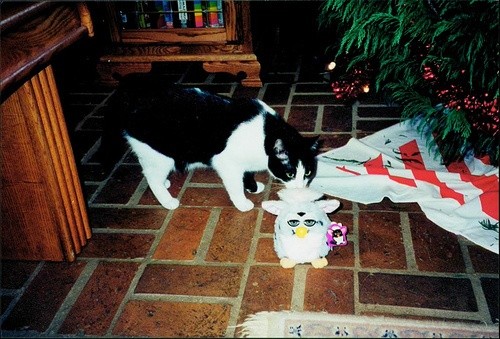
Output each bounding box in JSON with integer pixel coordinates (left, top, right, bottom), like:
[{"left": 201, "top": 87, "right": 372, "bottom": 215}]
[{"left": 1, "top": 1, "right": 97, "bottom": 263}]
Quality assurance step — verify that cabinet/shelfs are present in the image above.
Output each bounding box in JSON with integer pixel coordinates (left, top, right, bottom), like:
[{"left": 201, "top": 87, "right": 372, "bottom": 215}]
[{"left": 94, "top": 0, "right": 267, "bottom": 90}]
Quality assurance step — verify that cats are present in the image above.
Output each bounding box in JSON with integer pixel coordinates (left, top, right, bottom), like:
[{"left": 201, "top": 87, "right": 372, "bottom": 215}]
[{"left": 97, "top": 71, "right": 321, "bottom": 212}]
